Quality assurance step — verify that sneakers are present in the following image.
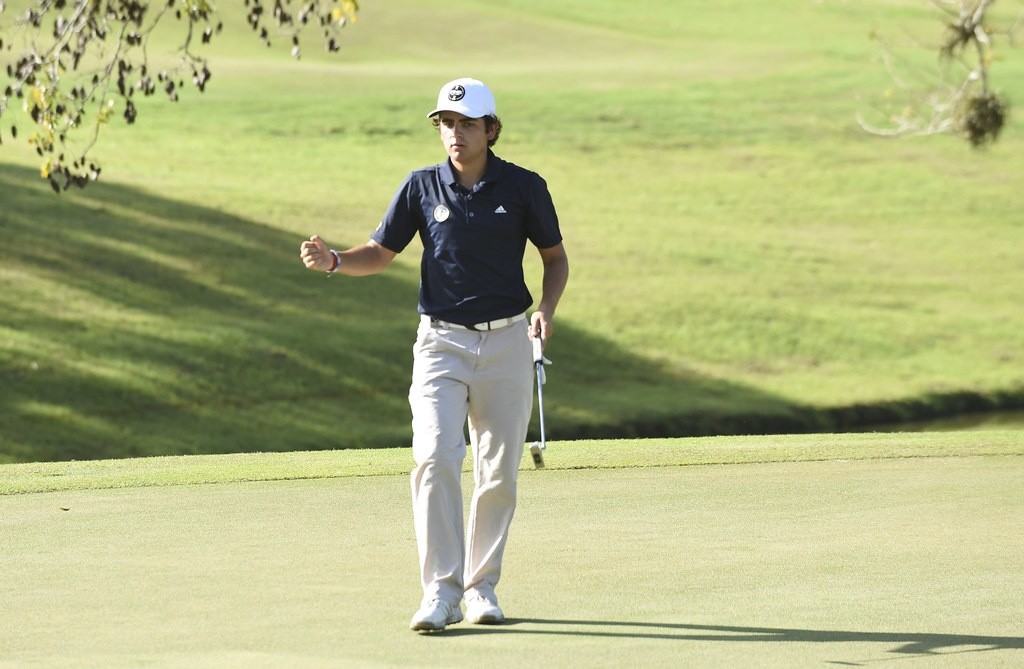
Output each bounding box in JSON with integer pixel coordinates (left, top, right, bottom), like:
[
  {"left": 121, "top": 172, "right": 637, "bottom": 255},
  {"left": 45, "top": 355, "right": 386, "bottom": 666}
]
[
  {"left": 464, "top": 581, "right": 504, "bottom": 625},
  {"left": 410, "top": 598, "right": 464, "bottom": 631}
]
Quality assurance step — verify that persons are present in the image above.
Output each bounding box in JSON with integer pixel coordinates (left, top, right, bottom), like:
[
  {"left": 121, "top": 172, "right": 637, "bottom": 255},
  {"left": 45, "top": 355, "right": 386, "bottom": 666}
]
[{"left": 299, "top": 77, "right": 572, "bottom": 632}]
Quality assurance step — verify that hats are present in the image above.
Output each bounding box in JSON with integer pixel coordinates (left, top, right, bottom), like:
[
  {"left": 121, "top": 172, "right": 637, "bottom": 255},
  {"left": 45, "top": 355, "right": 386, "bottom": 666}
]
[{"left": 428, "top": 78, "right": 496, "bottom": 120}]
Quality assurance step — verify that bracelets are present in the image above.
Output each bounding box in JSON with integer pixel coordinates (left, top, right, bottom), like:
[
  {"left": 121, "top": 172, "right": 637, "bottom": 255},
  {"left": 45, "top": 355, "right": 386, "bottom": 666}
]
[{"left": 325, "top": 249, "right": 341, "bottom": 277}]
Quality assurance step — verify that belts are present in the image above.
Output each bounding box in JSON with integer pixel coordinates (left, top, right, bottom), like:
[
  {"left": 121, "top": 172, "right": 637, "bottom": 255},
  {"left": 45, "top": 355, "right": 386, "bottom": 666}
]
[{"left": 419, "top": 310, "right": 527, "bottom": 330}]
